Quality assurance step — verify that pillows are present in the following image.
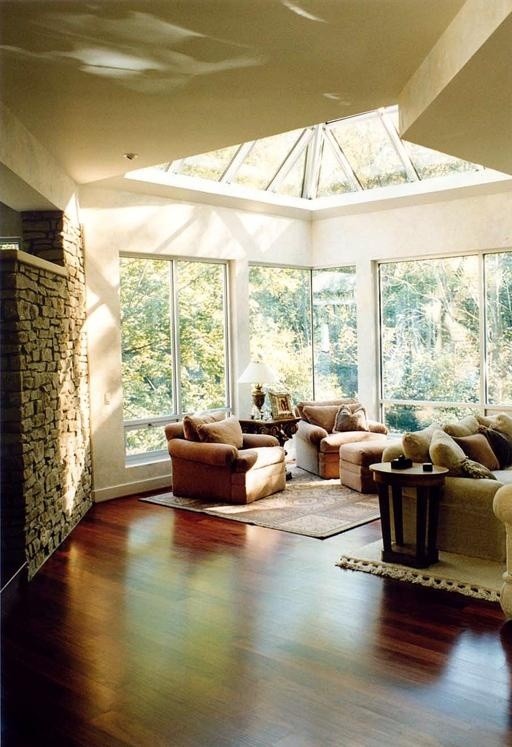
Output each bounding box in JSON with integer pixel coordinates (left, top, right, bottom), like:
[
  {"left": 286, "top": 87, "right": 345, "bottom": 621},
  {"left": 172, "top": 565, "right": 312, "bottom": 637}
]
[
  {"left": 182, "top": 412, "right": 227, "bottom": 441},
  {"left": 450, "top": 433, "right": 500, "bottom": 470},
  {"left": 458, "top": 415, "right": 480, "bottom": 434},
  {"left": 479, "top": 425, "right": 512, "bottom": 467},
  {"left": 402, "top": 422, "right": 444, "bottom": 465},
  {"left": 302, "top": 403, "right": 361, "bottom": 433},
  {"left": 332, "top": 404, "right": 370, "bottom": 433},
  {"left": 429, "top": 429, "right": 467, "bottom": 477},
  {"left": 197, "top": 416, "right": 243, "bottom": 450},
  {"left": 475, "top": 414, "right": 496, "bottom": 427},
  {"left": 442, "top": 422, "right": 474, "bottom": 438},
  {"left": 461, "top": 458, "right": 496, "bottom": 480}
]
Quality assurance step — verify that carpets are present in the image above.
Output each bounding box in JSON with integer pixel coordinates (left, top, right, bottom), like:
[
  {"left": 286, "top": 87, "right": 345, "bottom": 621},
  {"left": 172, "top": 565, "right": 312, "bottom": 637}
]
[
  {"left": 139, "top": 463, "right": 381, "bottom": 539},
  {"left": 335, "top": 535, "right": 512, "bottom": 604}
]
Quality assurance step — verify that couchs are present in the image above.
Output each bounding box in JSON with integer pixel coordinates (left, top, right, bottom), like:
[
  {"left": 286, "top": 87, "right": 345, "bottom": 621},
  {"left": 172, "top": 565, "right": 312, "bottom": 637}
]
[
  {"left": 339, "top": 440, "right": 393, "bottom": 493},
  {"left": 295, "top": 398, "right": 388, "bottom": 479},
  {"left": 381, "top": 413, "right": 512, "bottom": 562},
  {"left": 164, "top": 412, "right": 286, "bottom": 503}
]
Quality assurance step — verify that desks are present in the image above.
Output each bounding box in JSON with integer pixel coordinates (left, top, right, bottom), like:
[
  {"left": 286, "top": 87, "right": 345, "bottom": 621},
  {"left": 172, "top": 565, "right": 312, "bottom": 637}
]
[
  {"left": 236, "top": 416, "right": 300, "bottom": 481},
  {"left": 369, "top": 461, "right": 449, "bottom": 568}
]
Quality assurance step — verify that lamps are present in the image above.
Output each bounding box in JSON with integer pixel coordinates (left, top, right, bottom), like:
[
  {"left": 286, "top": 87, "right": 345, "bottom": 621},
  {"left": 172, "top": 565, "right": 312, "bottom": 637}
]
[{"left": 237, "top": 358, "right": 277, "bottom": 419}]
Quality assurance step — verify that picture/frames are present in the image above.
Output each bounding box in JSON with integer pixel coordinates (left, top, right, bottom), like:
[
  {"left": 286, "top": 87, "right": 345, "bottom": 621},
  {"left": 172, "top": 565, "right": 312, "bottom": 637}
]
[{"left": 268, "top": 391, "right": 296, "bottom": 419}]
